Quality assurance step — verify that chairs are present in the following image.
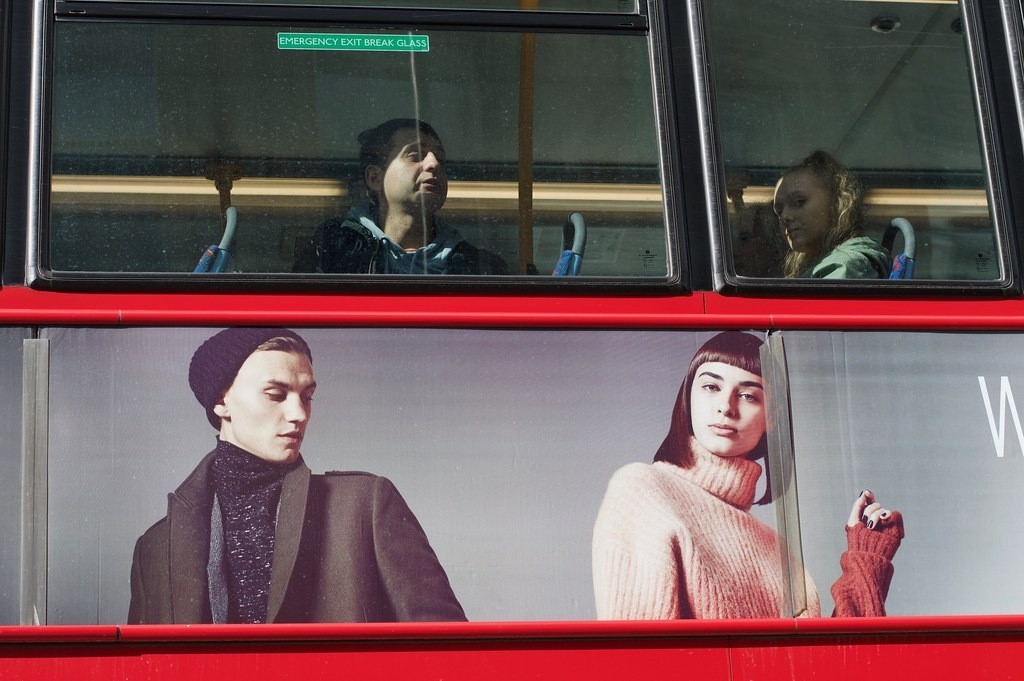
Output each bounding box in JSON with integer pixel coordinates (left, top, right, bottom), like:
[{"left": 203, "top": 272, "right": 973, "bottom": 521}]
[{"left": 191, "top": 209, "right": 918, "bottom": 285}]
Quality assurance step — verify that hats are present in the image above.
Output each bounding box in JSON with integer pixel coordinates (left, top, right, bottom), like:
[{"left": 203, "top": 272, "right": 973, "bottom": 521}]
[{"left": 188, "top": 325, "right": 315, "bottom": 430}]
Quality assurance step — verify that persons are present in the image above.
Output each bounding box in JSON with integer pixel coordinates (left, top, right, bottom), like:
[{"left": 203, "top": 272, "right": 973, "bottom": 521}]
[
  {"left": 127, "top": 327, "right": 469, "bottom": 619},
  {"left": 592, "top": 332, "right": 903, "bottom": 619},
  {"left": 773, "top": 150, "right": 890, "bottom": 275},
  {"left": 281, "top": 118, "right": 519, "bottom": 285}
]
